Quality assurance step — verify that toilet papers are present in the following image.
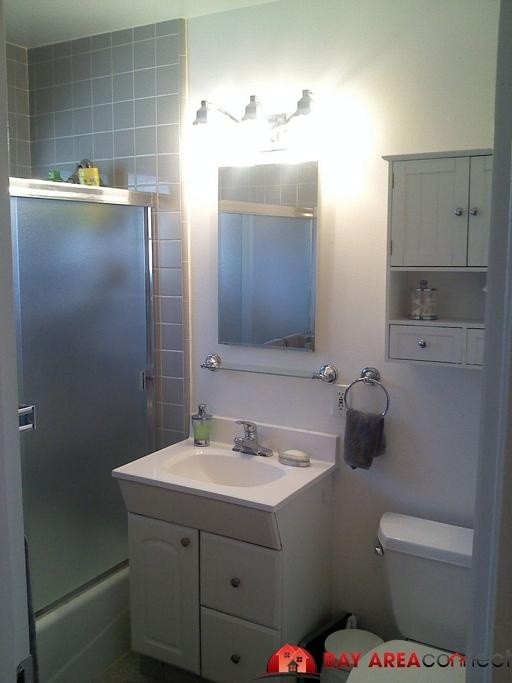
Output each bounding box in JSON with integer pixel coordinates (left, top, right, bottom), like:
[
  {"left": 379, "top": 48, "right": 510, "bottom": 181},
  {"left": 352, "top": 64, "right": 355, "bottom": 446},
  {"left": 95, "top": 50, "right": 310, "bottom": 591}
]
[
  {"left": 191, "top": 403, "right": 213, "bottom": 446},
  {"left": 279, "top": 449, "right": 311, "bottom": 466}
]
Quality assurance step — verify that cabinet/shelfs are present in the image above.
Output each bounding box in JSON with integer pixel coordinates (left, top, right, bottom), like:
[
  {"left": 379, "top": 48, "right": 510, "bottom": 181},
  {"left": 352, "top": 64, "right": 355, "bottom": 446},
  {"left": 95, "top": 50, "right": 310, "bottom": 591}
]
[
  {"left": 198, "top": 604, "right": 332, "bottom": 683},
  {"left": 197, "top": 531, "right": 335, "bottom": 630},
  {"left": 381, "top": 147, "right": 496, "bottom": 373},
  {"left": 124, "top": 512, "right": 200, "bottom": 678}
]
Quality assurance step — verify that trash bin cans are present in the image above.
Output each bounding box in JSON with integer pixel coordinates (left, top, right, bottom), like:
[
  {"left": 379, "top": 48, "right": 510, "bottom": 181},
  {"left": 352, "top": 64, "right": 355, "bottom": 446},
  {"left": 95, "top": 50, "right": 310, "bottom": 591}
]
[{"left": 323, "top": 629, "right": 385, "bottom": 683}]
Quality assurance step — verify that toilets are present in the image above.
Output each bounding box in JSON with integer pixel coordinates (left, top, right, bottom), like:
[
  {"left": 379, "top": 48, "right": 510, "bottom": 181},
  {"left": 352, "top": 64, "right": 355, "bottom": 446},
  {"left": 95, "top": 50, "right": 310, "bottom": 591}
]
[{"left": 345, "top": 511, "right": 473, "bottom": 683}]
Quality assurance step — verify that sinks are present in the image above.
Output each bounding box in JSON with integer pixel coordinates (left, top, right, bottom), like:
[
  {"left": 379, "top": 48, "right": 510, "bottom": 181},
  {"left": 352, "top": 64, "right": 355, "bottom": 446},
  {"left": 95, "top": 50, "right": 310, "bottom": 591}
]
[{"left": 162, "top": 452, "right": 288, "bottom": 488}]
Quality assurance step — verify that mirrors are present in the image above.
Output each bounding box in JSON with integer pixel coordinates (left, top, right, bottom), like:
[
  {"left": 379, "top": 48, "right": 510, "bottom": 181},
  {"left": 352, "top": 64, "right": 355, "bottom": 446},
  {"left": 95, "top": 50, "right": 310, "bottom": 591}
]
[{"left": 216, "top": 159, "right": 323, "bottom": 356}]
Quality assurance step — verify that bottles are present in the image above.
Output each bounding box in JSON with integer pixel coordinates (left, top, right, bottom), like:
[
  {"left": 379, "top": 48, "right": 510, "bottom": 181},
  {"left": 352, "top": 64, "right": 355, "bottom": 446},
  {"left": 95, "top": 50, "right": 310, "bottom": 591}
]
[{"left": 407, "top": 280, "right": 439, "bottom": 320}]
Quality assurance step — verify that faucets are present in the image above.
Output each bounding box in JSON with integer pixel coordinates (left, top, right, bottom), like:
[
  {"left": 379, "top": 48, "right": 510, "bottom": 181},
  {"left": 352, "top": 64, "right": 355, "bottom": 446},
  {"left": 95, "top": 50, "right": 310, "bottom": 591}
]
[{"left": 232, "top": 421, "right": 273, "bottom": 457}]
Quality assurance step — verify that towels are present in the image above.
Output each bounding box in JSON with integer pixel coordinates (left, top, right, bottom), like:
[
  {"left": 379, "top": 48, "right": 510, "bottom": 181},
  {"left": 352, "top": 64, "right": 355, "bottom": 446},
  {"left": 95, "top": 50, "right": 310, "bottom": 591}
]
[{"left": 341, "top": 408, "right": 388, "bottom": 470}]
